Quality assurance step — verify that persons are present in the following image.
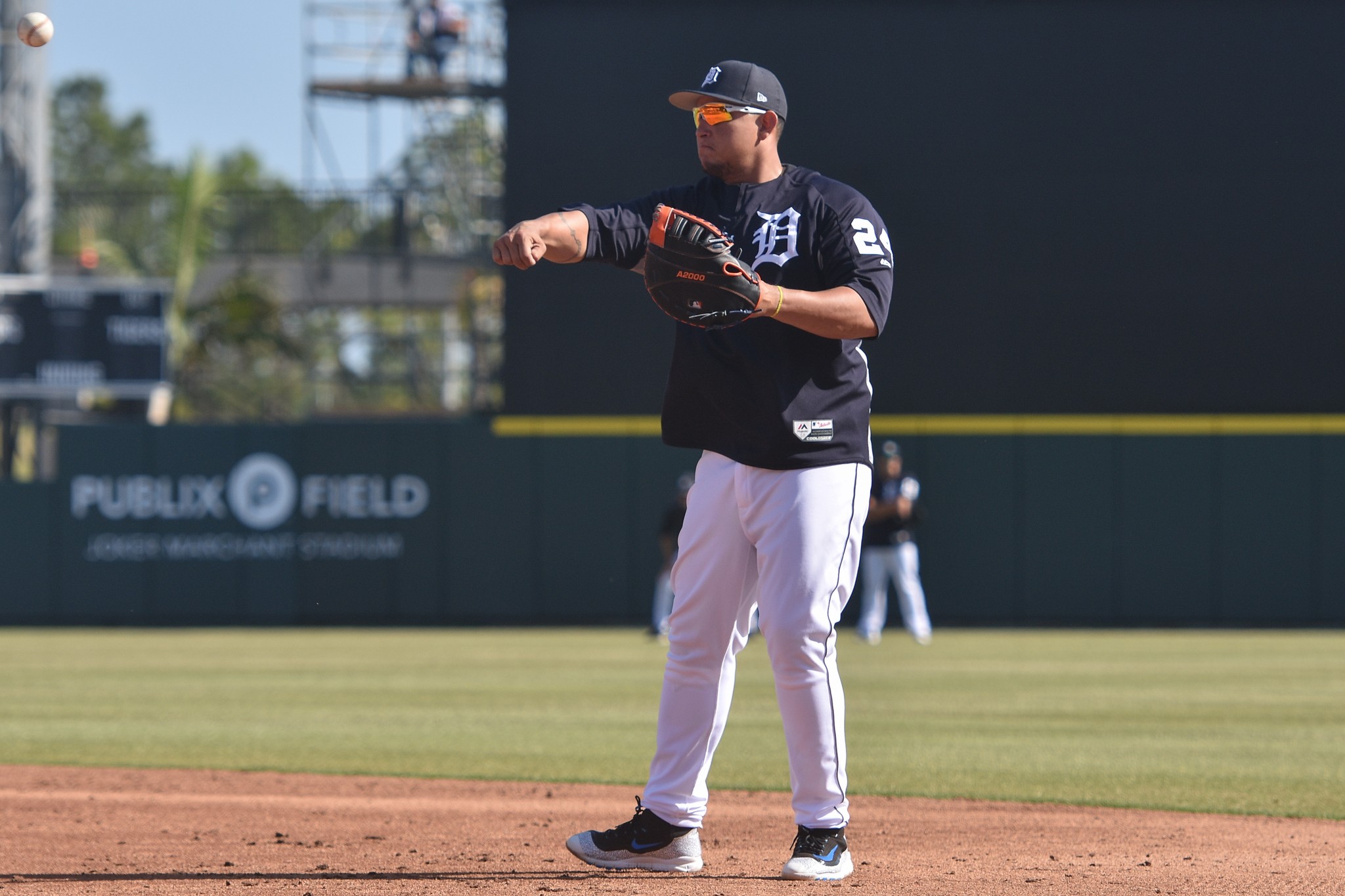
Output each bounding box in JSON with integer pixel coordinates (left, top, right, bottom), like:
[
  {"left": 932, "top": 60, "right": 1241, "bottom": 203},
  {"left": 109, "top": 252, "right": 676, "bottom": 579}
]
[
  {"left": 857, "top": 439, "right": 932, "bottom": 646},
  {"left": 650, "top": 470, "right": 695, "bottom": 637},
  {"left": 405, "top": 0, "right": 464, "bottom": 88},
  {"left": 494, "top": 59, "right": 895, "bottom": 881}
]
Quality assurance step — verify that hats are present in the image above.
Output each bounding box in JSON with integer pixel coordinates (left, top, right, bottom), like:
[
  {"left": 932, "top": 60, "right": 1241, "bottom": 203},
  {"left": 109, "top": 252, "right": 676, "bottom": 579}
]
[{"left": 668, "top": 60, "right": 786, "bottom": 127}]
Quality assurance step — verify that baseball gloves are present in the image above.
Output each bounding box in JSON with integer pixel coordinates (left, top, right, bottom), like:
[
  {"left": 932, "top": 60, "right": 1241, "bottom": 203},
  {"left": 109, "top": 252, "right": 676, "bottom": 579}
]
[{"left": 646, "top": 203, "right": 763, "bottom": 333}]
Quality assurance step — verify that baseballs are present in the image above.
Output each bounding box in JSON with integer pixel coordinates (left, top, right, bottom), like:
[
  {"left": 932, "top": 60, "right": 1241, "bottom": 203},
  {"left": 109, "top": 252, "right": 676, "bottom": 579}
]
[{"left": 18, "top": 10, "right": 50, "bottom": 44}]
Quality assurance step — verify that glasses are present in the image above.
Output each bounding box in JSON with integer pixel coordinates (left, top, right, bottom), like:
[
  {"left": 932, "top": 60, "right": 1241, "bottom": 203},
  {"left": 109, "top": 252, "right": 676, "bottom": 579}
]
[{"left": 692, "top": 102, "right": 767, "bottom": 130}]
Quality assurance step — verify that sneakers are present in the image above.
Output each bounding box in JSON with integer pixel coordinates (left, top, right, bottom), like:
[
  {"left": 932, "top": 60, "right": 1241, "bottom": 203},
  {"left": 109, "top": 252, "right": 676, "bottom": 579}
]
[
  {"left": 781, "top": 827, "right": 854, "bottom": 879},
  {"left": 565, "top": 795, "right": 704, "bottom": 873}
]
